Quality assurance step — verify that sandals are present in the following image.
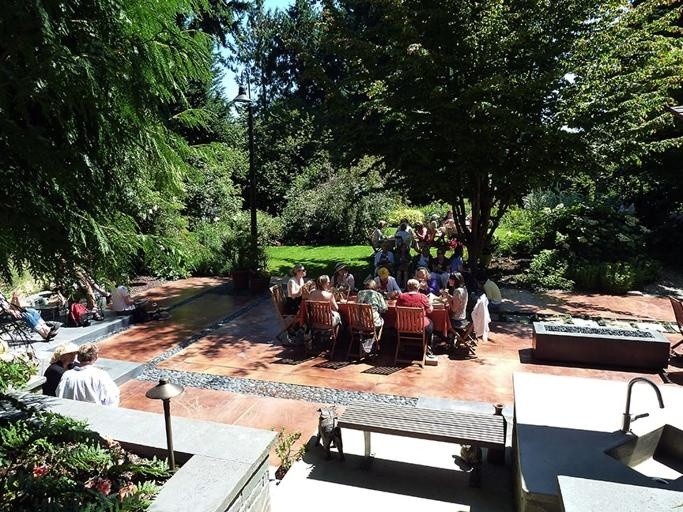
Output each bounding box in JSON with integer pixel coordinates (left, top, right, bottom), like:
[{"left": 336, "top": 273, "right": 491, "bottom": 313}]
[
  {"left": 90, "top": 305, "right": 99, "bottom": 314},
  {"left": 93, "top": 312, "right": 104, "bottom": 321}
]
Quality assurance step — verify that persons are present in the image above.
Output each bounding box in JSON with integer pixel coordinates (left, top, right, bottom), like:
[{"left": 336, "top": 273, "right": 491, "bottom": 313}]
[
  {"left": 368, "top": 211, "right": 462, "bottom": 298},
  {"left": 355, "top": 279, "right": 388, "bottom": 351},
  {"left": 42, "top": 344, "right": 80, "bottom": 396},
  {"left": 307, "top": 274, "right": 342, "bottom": 341},
  {"left": 473, "top": 269, "right": 502, "bottom": 309},
  {"left": 55, "top": 343, "right": 120, "bottom": 408},
  {"left": 113, "top": 273, "right": 139, "bottom": 317},
  {"left": 0, "top": 292, "right": 58, "bottom": 341},
  {"left": 394, "top": 279, "right": 434, "bottom": 358},
  {"left": 442, "top": 272, "right": 468, "bottom": 328},
  {"left": 284, "top": 263, "right": 307, "bottom": 316},
  {"left": 49, "top": 288, "right": 65, "bottom": 311},
  {"left": 330, "top": 263, "right": 355, "bottom": 293}
]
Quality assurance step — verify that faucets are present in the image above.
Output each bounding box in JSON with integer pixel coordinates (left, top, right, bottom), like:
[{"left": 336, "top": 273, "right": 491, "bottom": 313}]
[{"left": 621, "top": 377, "right": 665, "bottom": 432}]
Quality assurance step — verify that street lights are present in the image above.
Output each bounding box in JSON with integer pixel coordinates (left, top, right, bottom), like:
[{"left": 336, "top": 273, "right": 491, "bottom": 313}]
[
  {"left": 146, "top": 378, "right": 184, "bottom": 470},
  {"left": 234, "top": 69, "right": 256, "bottom": 265}
]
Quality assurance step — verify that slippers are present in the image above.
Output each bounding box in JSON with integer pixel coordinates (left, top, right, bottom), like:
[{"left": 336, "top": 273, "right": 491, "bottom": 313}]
[
  {"left": 51, "top": 323, "right": 60, "bottom": 331},
  {"left": 46, "top": 331, "right": 58, "bottom": 341},
  {"left": 100, "top": 292, "right": 112, "bottom": 297}
]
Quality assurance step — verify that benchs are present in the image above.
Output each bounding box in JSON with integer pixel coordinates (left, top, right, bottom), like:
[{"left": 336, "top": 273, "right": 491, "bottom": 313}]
[{"left": 334, "top": 401, "right": 505, "bottom": 482}]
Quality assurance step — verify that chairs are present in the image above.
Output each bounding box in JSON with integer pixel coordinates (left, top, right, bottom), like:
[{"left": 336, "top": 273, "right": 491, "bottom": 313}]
[
  {"left": 667, "top": 294, "right": 683, "bottom": 351},
  {"left": 268, "top": 283, "right": 305, "bottom": 346},
  {"left": 303, "top": 299, "right": 336, "bottom": 360},
  {"left": 337, "top": 301, "right": 382, "bottom": 365},
  {"left": 393, "top": 305, "right": 428, "bottom": 369},
  {"left": 0, "top": 288, "right": 64, "bottom": 345},
  {"left": 365, "top": 231, "right": 453, "bottom": 260},
  {"left": 449, "top": 292, "right": 487, "bottom": 356}
]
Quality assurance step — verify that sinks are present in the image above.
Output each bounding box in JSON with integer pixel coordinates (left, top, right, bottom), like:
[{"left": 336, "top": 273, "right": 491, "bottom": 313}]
[{"left": 603, "top": 423, "right": 683, "bottom": 483}]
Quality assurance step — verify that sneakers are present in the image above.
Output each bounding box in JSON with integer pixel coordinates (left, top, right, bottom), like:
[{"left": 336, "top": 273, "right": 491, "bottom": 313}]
[{"left": 427, "top": 347, "right": 433, "bottom": 356}]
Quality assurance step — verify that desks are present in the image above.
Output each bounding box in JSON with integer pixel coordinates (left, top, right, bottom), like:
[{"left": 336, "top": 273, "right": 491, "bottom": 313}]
[{"left": 29, "top": 301, "right": 59, "bottom": 322}]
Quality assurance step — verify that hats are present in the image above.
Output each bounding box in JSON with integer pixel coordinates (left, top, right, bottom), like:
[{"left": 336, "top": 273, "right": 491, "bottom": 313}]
[
  {"left": 60, "top": 343, "right": 80, "bottom": 356},
  {"left": 333, "top": 262, "right": 348, "bottom": 274}
]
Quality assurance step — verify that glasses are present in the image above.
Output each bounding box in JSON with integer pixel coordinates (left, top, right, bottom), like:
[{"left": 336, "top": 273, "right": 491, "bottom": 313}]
[
  {"left": 416, "top": 268, "right": 424, "bottom": 271},
  {"left": 298, "top": 269, "right": 305, "bottom": 272}
]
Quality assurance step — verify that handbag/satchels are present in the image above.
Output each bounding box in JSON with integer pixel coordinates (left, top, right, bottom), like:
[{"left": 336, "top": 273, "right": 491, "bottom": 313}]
[
  {"left": 276, "top": 329, "right": 296, "bottom": 347},
  {"left": 11, "top": 287, "right": 26, "bottom": 308},
  {"left": 302, "top": 280, "right": 317, "bottom": 300}
]
